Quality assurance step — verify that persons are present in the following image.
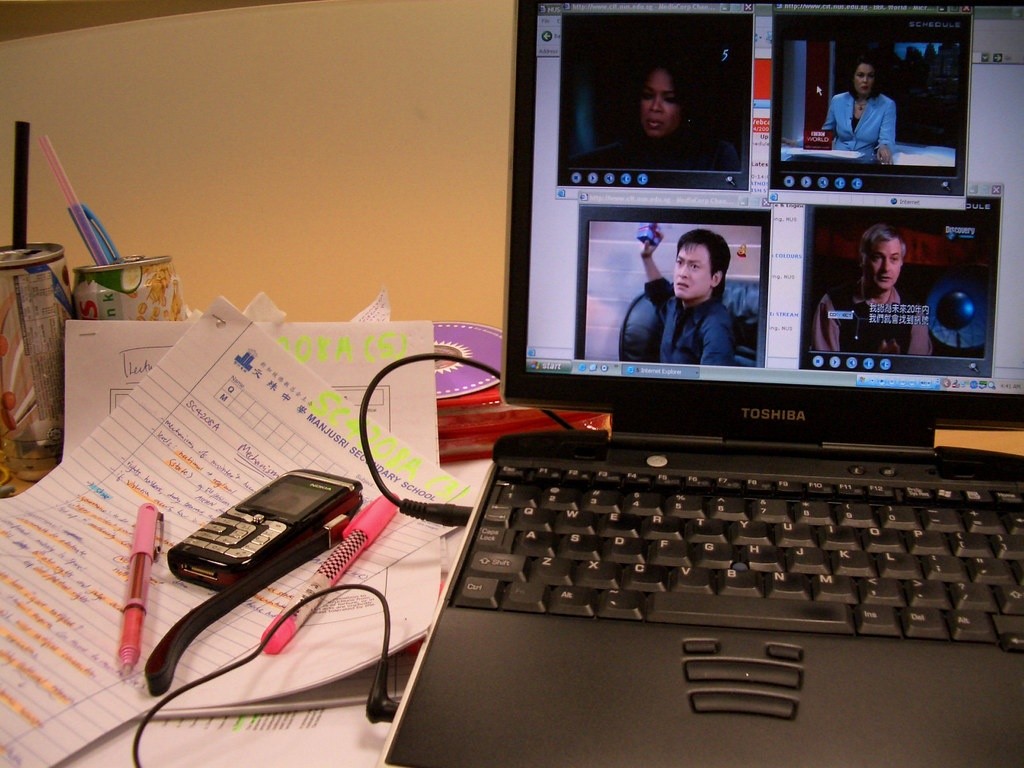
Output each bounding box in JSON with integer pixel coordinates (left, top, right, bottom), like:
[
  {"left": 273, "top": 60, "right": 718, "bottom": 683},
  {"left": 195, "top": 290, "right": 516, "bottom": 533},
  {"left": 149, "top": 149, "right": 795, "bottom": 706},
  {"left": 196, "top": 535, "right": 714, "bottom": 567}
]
[
  {"left": 782, "top": 58, "right": 896, "bottom": 164},
  {"left": 566, "top": 61, "right": 740, "bottom": 172},
  {"left": 641, "top": 225, "right": 735, "bottom": 366},
  {"left": 813, "top": 224, "right": 935, "bottom": 355}
]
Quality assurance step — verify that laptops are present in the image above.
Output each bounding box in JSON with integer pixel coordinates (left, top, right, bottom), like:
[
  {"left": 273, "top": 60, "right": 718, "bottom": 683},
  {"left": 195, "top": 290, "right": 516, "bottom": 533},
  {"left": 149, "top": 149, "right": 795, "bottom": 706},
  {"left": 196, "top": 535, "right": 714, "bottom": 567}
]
[{"left": 386, "top": 0, "right": 1024, "bottom": 768}]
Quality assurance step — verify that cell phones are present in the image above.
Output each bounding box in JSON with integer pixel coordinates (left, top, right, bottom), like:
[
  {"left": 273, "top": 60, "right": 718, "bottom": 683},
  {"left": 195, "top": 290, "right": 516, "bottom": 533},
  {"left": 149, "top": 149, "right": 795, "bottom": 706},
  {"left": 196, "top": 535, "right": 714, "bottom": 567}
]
[{"left": 168, "top": 469, "right": 361, "bottom": 593}]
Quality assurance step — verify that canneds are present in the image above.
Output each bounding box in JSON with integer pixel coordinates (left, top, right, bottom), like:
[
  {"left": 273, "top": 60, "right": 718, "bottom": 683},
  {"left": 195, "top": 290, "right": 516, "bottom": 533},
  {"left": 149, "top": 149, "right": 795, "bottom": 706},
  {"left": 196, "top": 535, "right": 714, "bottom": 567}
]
[
  {"left": 0, "top": 242, "right": 76, "bottom": 481},
  {"left": 73, "top": 254, "right": 188, "bottom": 321}
]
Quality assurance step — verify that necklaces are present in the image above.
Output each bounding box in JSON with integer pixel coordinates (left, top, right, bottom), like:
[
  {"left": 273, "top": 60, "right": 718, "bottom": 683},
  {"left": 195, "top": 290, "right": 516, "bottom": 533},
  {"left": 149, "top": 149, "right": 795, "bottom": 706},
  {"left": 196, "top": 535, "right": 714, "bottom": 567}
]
[{"left": 855, "top": 102, "right": 865, "bottom": 112}]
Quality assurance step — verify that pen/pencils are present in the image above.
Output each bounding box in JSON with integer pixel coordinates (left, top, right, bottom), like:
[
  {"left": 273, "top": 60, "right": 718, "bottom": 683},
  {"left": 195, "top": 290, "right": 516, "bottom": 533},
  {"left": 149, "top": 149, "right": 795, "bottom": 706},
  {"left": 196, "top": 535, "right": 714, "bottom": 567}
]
[
  {"left": 118, "top": 503, "right": 164, "bottom": 673},
  {"left": 67, "top": 205, "right": 120, "bottom": 264},
  {"left": 262, "top": 491, "right": 401, "bottom": 653}
]
